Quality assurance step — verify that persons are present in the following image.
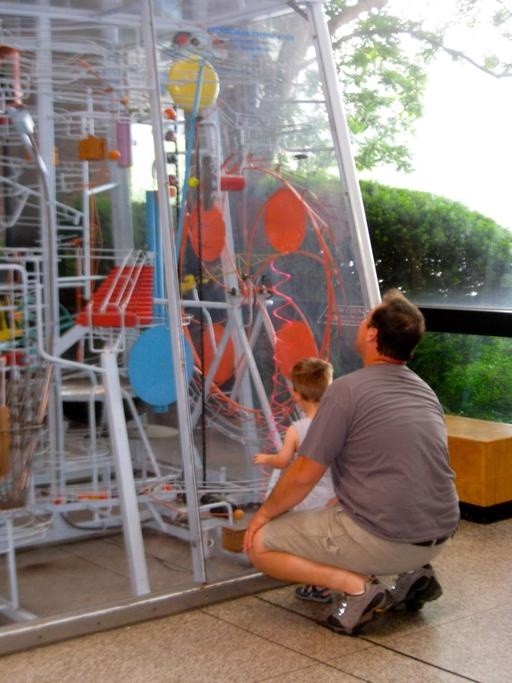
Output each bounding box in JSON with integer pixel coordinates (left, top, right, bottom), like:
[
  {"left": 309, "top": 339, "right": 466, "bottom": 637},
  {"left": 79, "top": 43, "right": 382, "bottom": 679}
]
[
  {"left": 241, "top": 290, "right": 461, "bottom": 638},
  {"left": 251, "top": 356, "right": 339, "bottom": 604}
]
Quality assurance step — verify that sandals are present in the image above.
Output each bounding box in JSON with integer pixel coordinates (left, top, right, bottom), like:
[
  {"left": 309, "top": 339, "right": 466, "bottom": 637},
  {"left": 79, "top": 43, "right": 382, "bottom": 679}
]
[{"left": 295, "top": 584, "right": 332, "bottom": 603}]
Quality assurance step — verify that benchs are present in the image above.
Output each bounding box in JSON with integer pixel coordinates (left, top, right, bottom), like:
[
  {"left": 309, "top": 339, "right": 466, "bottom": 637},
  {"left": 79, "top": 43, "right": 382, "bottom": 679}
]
[{"left": 444, "top": 415, "right": 512, "bottom": 525}]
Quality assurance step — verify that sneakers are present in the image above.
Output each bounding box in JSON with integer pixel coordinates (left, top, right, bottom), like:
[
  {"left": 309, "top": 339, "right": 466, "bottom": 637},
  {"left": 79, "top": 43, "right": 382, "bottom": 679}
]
[
  {"left": 327, "top": 573, "right": 395, "bottom": 635},
  {"left": 388, "top": 564, "right": 442, "bottom": 611}
]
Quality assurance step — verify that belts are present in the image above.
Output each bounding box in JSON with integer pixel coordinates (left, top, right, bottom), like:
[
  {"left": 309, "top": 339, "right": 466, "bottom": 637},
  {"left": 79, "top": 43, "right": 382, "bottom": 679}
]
[{"left": 412, "top": 536, "right": 450, "bottom": 546}]
